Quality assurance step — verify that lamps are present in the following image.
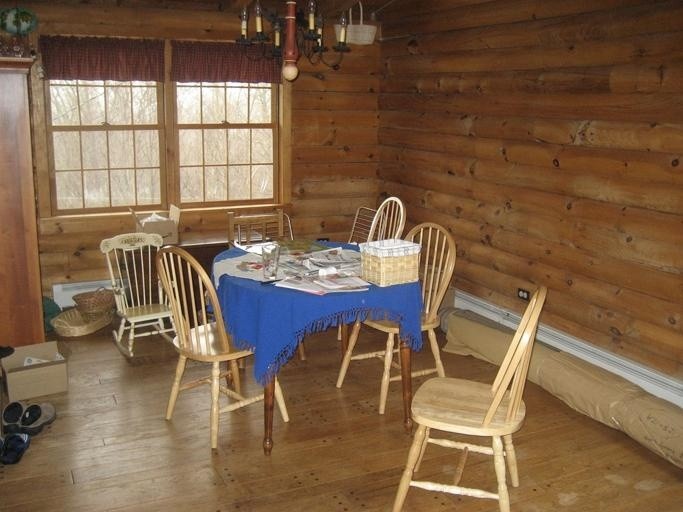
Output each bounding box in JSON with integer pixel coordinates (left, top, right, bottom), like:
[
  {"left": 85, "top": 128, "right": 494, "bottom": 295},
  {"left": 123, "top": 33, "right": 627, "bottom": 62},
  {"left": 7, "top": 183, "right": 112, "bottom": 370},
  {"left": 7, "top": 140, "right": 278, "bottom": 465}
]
[{"left": 234, "top": 0, "right": 352, "bottom": 82}]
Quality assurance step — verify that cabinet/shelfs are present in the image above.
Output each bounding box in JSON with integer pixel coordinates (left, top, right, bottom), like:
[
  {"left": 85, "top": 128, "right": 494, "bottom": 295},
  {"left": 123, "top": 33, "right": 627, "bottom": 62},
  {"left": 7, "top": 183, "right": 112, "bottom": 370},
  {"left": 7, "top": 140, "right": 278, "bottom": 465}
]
[
  {"left": 0, "top": 53, "right": 45, "bottom": 348},
  {"left": 128, "top": 230, "right": 272, "bottom": 315}
]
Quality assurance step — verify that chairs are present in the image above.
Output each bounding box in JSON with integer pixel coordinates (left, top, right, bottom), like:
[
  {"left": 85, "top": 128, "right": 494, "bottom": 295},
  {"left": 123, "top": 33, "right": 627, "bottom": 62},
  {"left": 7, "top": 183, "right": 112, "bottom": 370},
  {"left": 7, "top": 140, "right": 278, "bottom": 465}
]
[
  {"left": 394, "top": 285, "right": 549, "bottom": 512},
  {"left": 100, "top": 233, "right": 177, "bottom": 358},
  {"left": 228, "top": 208, "right": 284, "bottom": 248},
  {"left": 155, "top": 246, "right": 290, "bottom": 448},
  {"left": 367, "top": 197, "right": 406, "bottom": 240},
  {"left": 337, "top": 222, "right": 457, "bottom": 415}
]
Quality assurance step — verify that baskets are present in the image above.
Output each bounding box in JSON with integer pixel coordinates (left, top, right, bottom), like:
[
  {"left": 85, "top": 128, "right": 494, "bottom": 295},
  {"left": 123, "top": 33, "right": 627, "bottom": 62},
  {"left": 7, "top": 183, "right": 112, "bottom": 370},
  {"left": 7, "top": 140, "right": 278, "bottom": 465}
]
[{"left": 49, "top": 290, "right": 113, "bottom": 337}]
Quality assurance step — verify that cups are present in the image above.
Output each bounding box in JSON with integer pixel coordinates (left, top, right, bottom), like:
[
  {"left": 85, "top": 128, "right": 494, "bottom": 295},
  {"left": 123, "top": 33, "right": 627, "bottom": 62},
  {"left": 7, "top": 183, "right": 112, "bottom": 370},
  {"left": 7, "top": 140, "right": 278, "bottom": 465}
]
[{"left": 262, "top": 243, "right": 281, "bottom": 280}]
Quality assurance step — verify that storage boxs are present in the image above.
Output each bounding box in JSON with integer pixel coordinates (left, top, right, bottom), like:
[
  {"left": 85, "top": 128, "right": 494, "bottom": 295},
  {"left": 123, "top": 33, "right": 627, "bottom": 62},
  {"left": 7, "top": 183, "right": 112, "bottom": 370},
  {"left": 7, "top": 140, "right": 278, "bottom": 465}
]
[
  {"left": 0, "top": 340, "right": 68, "bottom": 403},
  {"left": 127, "top": 203, "right": 181, "bottom": 244}
]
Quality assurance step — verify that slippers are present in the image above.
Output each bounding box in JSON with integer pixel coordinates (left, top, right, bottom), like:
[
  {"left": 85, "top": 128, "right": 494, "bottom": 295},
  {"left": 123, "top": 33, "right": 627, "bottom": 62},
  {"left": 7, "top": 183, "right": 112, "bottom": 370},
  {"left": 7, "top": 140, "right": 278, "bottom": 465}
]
[{"left": 0, "top": 401, "right": 56, "bottom": 465}]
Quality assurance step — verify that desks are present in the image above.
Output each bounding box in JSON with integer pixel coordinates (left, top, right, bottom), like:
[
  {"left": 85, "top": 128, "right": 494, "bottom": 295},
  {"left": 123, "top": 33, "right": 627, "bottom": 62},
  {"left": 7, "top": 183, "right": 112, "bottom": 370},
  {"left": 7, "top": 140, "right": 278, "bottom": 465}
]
[{"left": 206, "top": 241, "right": 426, "bottom": 456}]
